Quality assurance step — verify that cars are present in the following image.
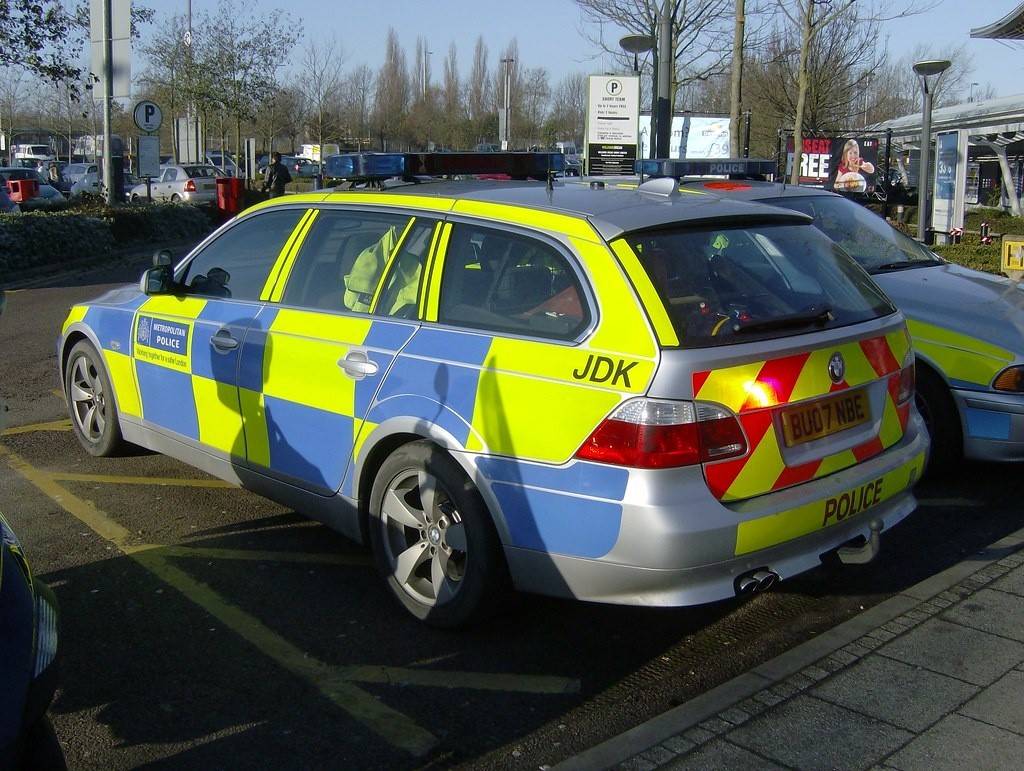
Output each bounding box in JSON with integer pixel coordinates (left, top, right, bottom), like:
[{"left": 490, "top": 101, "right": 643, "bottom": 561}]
[
  {"left": 347, "top": 140, "right": 583, "bottom": 188},
  {"left": 0, "top": 143, "right": 326, "bottom": 216},
  {"left": 512, "top": 158, "right": 1024, "bottom": 500},
  {"left": 55, "top": 149, "right": 934, "bottom": 636}
]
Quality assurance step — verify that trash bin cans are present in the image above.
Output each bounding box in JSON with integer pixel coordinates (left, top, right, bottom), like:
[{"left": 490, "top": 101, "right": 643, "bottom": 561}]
[
  {"left": 215, "top": 177, "right": 246, "bottom": 228},
  {"left": 6, "top": 179, "right": 40, "bottom": 202},
  {"left": 312, "top": 174, "right": 323, "bottom": 191}
]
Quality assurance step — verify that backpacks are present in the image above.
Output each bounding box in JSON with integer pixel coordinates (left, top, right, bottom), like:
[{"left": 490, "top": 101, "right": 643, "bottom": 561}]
[{"left": 270, "top": 170, "right": 285, "bottom": 196}]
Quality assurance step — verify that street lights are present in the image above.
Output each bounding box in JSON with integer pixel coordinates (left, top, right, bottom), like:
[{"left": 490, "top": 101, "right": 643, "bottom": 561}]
[
  {"left": 911, "top": 60, "right": 952, "bottom": 245},
  {"left": 500, "top": 58, "right": 514, "bottom": 150},
  {"left": 617, "top": 32, "right": 655, "bottom": 77},
  {"left": 423, "top": 49, "right": 434, "bottom": 102}
]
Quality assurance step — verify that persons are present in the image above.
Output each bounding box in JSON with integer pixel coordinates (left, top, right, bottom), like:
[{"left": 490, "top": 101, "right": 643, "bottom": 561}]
[
  {"left": 47, "top": 163, "right": 64, "bottom": 192},
  {"left": 238, "top": 154, "right": 245, "bottom": 171},
  {"left": 37, "top": 161, "right": 45, "bottom": 173},
  {"left": 833, "top": 139, "right": 874, "bottom": 189},
  {"left": 261, "top": 151, "right": 292, "bottom": 198}
]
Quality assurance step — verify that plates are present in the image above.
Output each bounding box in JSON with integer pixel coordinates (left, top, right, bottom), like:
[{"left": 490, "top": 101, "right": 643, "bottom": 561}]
[{"left": 840, "top": 172, "right": 866, "bottom": 193}]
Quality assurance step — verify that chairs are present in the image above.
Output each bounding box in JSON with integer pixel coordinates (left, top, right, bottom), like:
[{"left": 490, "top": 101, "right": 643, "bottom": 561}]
[{"left": 316, "top": 233, "right": 384, "bottom": 309}]
